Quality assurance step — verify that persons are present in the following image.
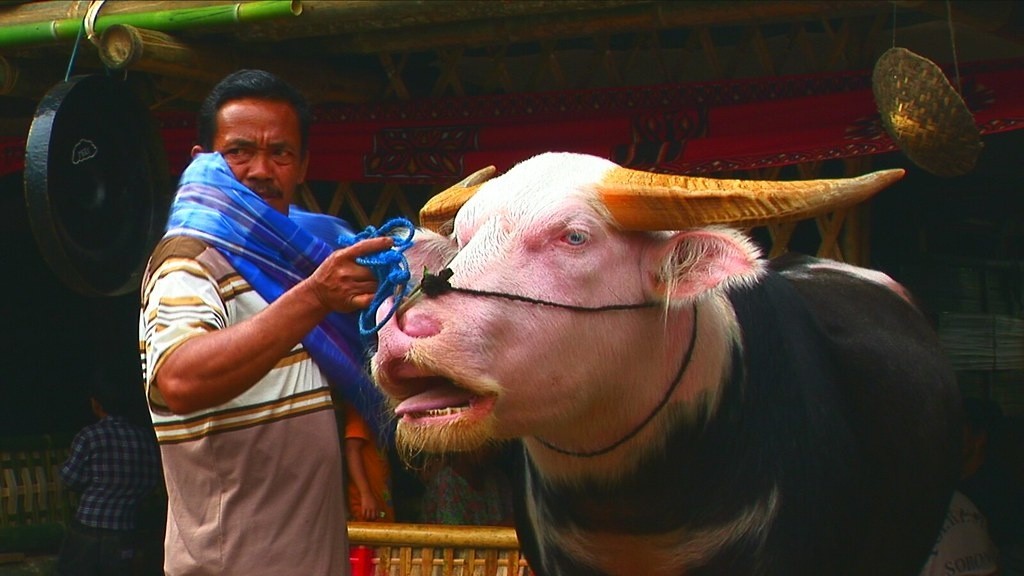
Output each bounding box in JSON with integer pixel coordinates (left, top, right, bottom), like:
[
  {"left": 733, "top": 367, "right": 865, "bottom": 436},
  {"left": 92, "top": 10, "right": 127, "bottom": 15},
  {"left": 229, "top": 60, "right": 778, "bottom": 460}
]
[
  {"left": 54, "top": 369, "right": 158, "bottom": 576},
  {"left": 344, "top": 405, "right": 504, "bottom": 527},
  {"left": 919, "top": 398, "right": 1024, "bottom": 576},
  {"left": 139, "top": 69, "right": 393, "bottom": 576}
]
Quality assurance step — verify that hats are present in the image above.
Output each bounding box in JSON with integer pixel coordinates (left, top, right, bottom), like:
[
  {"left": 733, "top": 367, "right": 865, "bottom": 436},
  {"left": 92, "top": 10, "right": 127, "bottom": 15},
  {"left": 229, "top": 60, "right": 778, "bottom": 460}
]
[{"left": 871, "top": 47, "right": 983, "bottom": 179}]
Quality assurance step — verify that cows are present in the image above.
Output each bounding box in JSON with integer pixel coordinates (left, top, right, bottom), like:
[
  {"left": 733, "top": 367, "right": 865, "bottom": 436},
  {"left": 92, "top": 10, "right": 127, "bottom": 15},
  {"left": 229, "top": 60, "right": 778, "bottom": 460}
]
[{"left": 359, "top": 141, "right": 1010, "bottom": 576}]
